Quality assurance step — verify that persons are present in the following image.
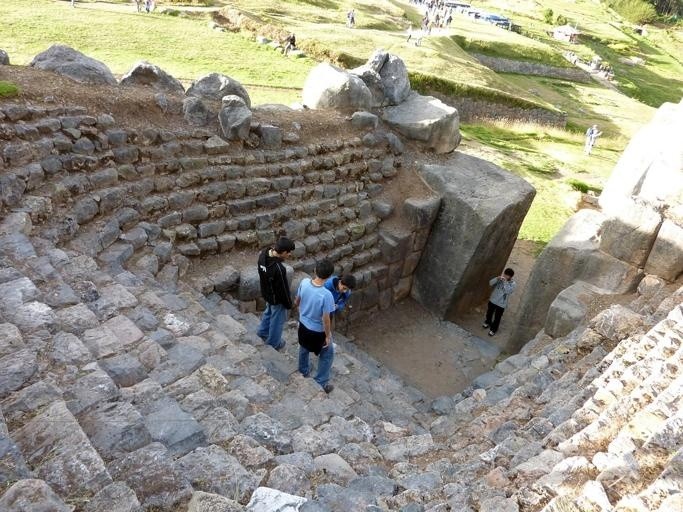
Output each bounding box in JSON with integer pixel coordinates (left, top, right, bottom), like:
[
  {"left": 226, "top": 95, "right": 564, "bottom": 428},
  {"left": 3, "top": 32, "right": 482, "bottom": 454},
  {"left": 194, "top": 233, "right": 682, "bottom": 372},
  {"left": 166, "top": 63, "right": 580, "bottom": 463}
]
[
  {"left": 135, "top": 0, "right": 156, "bottom": 14},
  {"left": 347, "top": 9, "right": 355, "bottom": 29},
  {"left": 294, "top": 259, "right": 336, "bottom": 393},
  {"left": 482, "top": 268, "right": 516, "bottom": 336},
  {"left": 256, "top": 237, "right": 295, "bottom": 350},
  {"left": 324, "top": 274, "right": 356, "bottom": 322},
  {"left": 281, "top": 32, "right": 295, "bottom": 55},
  {"left": 584, "top": 124, "right": 603, "bottom": 156}
]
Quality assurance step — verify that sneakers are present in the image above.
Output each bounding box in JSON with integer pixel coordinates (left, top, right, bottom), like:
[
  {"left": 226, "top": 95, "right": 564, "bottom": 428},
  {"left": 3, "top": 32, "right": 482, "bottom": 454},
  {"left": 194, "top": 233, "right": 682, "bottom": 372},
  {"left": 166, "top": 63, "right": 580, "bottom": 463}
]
[
  {"left": 482, "top": 322, "right": 496, "bottom": 336},
  {"left": 275, "top": 340, "right": 286, "bottom": 351},
  {"left": 324, "top": 385, "right": 334, "bottom": 393}
]
